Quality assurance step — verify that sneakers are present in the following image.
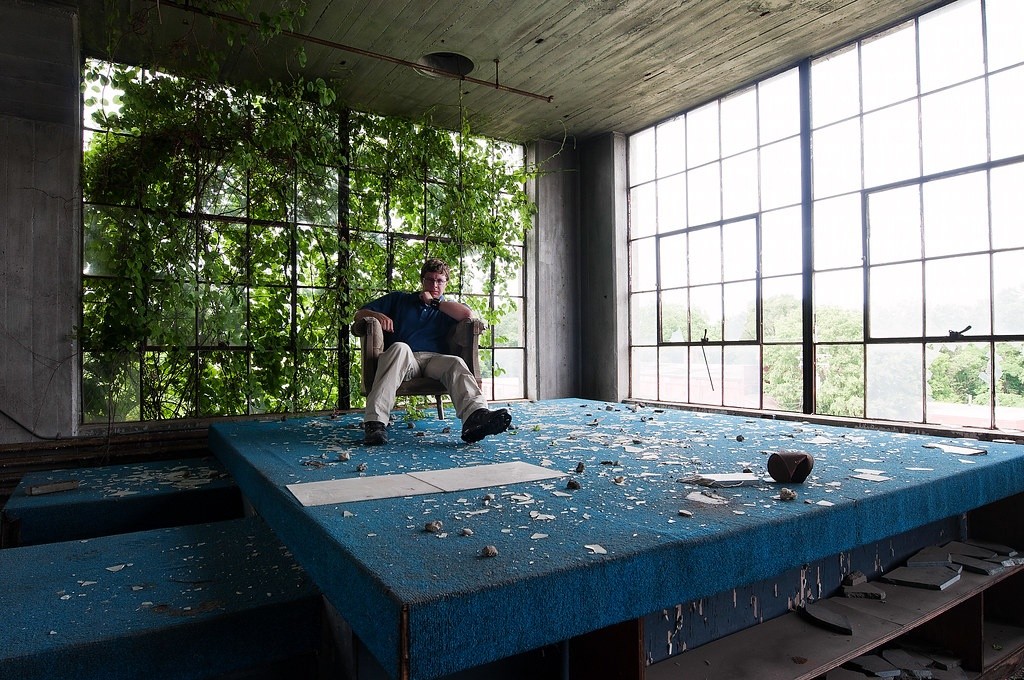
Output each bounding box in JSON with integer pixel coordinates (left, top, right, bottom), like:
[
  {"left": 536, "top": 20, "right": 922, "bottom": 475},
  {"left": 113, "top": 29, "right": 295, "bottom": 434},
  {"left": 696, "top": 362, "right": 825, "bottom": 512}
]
[
  {"left": 363, "top": 420, "right": 389, "bottom": 445},
  {"left": 461, "top": 409, "right": 512, "bottom": 444}
]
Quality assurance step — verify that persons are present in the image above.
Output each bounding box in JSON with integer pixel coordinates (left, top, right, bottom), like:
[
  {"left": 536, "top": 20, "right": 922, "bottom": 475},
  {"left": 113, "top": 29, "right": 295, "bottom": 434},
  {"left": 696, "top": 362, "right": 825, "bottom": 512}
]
[{"left": 354, "top": 258, "right": 512, "bottom": 446}]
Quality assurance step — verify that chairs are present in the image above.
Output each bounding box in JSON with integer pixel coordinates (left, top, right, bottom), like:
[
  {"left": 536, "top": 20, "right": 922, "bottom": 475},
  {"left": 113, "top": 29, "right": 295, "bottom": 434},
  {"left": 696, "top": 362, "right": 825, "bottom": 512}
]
[{"left": 351, "top": 317, "right": 486, "bottom": 420}]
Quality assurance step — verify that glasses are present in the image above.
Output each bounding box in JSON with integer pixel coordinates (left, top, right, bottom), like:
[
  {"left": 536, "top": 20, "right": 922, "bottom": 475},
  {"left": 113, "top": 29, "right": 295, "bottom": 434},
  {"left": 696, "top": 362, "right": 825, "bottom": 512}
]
[{"left": 421, "top": 276, "right": 449, "bottom": 285}]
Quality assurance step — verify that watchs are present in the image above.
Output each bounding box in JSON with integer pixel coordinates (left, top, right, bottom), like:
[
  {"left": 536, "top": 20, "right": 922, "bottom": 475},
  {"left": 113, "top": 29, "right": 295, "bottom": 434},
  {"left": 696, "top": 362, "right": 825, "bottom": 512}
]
[{"left": 430, "top": 299, "right": 441, "bottom": 310}]
